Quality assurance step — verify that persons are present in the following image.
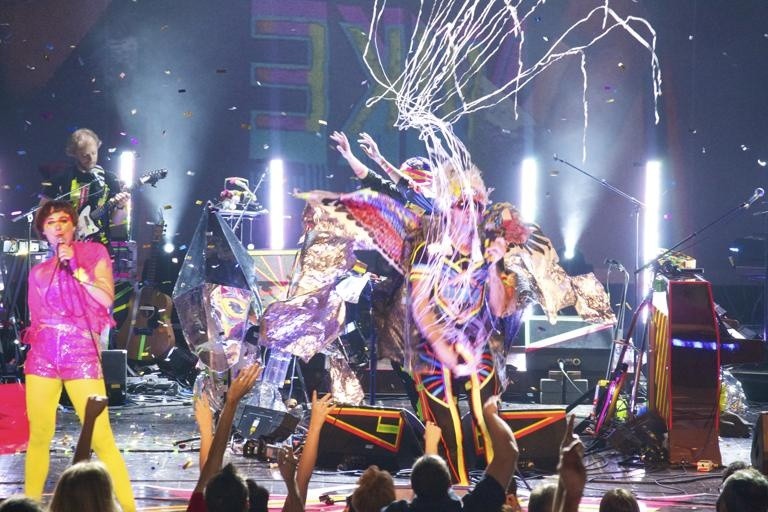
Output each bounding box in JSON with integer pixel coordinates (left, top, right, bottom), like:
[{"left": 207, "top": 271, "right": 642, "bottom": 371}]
[
  {"left": 21, "top": 198, "right": 141, "bottom": 510},
  {"left": 326, "top": 130, "right": 518, "bottom": 499},
  {"left": 0, "top": 363, "right": 336, "bottom": 511},
  {"left": 715, "top": 461, "right": 766, "bottom": 512},
  {"left": 33, "top": 125, "right": 129, "bottom": 241},
  {"left": 349, "top": 392, "right": 642, "bottom": 511}
]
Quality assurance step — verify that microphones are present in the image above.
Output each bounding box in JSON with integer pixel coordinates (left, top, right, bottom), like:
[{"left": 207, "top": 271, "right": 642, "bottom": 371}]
[
  {"left": 604, "top": 257, "right": 625, "bottom": 272},
  {"left": 55, "top": 237, "right": 69, "bottom": 267},
  {"left": 89, "top": 169, "right": 105, "bottom": 187},
  {"left": 744, "top": 188, "right": 764, "bottom": 208}
]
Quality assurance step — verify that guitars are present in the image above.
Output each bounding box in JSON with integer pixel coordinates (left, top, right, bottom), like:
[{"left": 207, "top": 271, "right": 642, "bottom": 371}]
[
  {"left": 78, "top": 169, "right": 166, "bottom": 241},
  {"left": 116, "top": 211, "right": 176, "bottom": 363},
  {"left": 592, "top": 290, "right": 653, "bottom": 436}
]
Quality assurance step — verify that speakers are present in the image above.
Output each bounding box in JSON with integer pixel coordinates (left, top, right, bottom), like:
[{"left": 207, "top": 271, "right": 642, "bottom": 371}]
[
  {"left": 461, "top": 409, "right": 566, "bottom": 474},
  {"left": 316, "top": 406, "right": 425, "bottom": 472},
  {"left": 102, "top": 350, "right": 127, "bottom": 405},
  {"left": 525, "top": 314, "right": 615, "bottom": 381}
]
